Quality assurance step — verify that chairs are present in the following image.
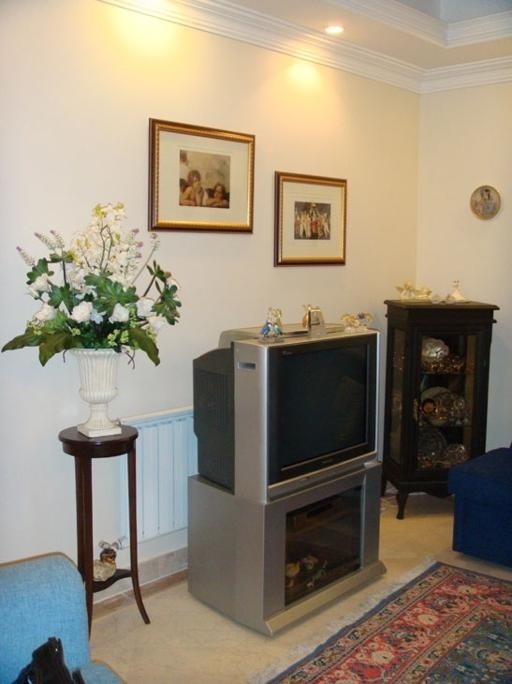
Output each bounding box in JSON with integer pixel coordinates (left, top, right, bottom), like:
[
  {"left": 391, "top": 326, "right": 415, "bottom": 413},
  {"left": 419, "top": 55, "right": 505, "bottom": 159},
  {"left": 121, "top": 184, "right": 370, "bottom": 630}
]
[{"left": 0, "top": 550, "right": 126, "bottom": 683}]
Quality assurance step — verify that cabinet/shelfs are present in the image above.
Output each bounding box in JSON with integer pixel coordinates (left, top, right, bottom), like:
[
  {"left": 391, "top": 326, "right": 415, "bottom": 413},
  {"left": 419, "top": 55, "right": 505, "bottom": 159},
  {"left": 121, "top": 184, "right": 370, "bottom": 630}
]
[
  {"left": 183, "top": 460, "right": 387, "bottom": 636},
  {"left": 381, "top": 297, "right": 502, "bottom": 527}
]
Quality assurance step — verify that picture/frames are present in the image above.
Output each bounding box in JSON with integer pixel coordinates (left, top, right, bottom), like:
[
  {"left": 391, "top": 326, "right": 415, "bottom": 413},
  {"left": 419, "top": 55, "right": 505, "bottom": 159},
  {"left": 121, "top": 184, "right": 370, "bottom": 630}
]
[
  {"left": 469, "top": 183, "right": 504, "bottom": 221},
  {"left": 272, "top": 169, "right": 349, "bottom": 268},
  {"left": 144, "top": 114, "right": 258, "bottom": 238}
]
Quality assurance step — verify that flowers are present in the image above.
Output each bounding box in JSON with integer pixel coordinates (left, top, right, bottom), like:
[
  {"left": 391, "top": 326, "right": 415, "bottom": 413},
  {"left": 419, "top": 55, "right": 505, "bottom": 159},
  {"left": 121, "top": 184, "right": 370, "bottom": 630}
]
[{"left": 2, "top": 194, "right": 184, "bottom": 368}]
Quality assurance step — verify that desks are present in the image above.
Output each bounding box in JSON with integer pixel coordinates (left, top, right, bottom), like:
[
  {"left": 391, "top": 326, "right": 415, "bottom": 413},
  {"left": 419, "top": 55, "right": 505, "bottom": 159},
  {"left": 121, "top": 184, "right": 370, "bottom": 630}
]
[{"left": 56, "top": 425, "right": 156, "bottom": 638}]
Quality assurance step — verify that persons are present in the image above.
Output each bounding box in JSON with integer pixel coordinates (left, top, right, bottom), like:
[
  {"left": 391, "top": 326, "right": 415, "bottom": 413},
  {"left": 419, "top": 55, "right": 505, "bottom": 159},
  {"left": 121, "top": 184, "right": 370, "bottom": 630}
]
[
  {"left": 294, "top": 209, "right": 330, "bottom": 240},
  {"left": 180, "top": 169, "right": 204, "bottom": 206},
  {"left": 203, "top": 181, "right": 229, "bottom": 208}
]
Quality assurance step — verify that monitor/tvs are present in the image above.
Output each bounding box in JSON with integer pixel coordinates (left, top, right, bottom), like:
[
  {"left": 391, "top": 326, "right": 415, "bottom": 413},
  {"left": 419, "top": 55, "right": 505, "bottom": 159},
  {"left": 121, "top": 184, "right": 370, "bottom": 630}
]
[{"left": 193, "top": 323, "right": 381, "bottom": 504}]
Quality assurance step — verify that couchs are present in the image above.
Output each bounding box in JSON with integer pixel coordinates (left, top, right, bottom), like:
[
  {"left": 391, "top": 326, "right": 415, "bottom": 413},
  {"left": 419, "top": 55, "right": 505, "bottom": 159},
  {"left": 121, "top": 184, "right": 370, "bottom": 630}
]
[{"left": 449, "top": 442, "right": 512, "bottom": 566}]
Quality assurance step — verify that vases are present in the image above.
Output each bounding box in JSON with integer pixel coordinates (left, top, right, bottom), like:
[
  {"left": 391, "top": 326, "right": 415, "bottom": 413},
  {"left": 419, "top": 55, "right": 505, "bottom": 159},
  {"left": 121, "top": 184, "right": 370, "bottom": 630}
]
[{"left": 69, "top": 341, "right": 126, "bottom": 439}]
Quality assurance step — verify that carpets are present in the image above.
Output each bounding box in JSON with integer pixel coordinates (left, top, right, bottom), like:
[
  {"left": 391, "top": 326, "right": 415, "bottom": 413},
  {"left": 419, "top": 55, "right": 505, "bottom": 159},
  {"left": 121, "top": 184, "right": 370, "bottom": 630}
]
[{"left": 270, "top": 558, "right": 511, "bottom": 681}]
